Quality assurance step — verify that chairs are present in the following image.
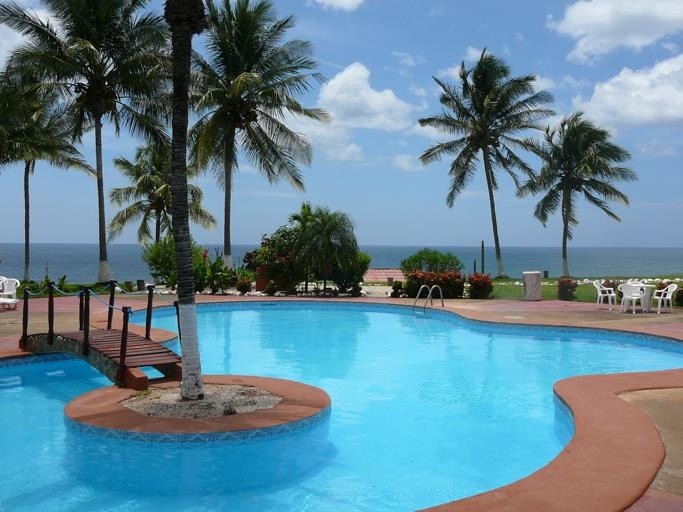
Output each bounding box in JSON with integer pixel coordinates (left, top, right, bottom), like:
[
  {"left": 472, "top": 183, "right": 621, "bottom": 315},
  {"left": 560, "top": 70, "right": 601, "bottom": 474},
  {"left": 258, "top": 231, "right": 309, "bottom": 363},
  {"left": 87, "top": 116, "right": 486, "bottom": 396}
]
[
  {"left": 592, "top": 279, "right": 678, "bottom": 315},
  {"left": 0, "top": 276, "right": 20, "bottom": 310}
]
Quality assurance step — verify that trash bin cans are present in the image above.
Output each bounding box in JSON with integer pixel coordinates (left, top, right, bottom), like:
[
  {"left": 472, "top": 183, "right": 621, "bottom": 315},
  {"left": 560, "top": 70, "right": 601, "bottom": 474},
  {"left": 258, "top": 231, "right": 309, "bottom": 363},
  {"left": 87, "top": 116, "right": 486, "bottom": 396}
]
[
  {"left": 387, "top": 277, "right": 393, "bottom": 286},
  {"left": 124, "top": 281, "right": 132, "bottom": 289},
  {"left": 543, "top": 270, "right": 549, "bottom": 279},
  {"left": 255, "top": 267, "right": 266, "bottom": 291},
  {"left": 559, "top": 277, "right": 574, "bottom": 301}
]
